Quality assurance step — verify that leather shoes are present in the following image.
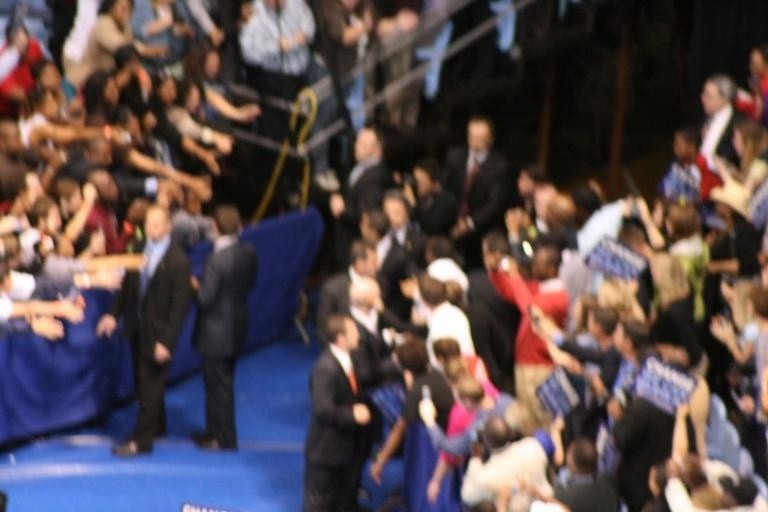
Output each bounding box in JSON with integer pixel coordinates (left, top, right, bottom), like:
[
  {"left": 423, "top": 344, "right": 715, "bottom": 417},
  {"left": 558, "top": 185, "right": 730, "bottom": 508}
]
[
  {"left": 111, "top": 441, "right": 152, "bottom": 456},
  {"left": 192, "top": 433, "right": 237, "bottom": 449}
]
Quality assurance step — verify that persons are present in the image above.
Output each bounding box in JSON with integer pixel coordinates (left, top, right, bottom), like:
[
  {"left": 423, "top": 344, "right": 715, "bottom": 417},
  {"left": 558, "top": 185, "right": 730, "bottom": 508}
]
[{"left": 0, "top": 0, "right": 767, "bottom": 512}]
